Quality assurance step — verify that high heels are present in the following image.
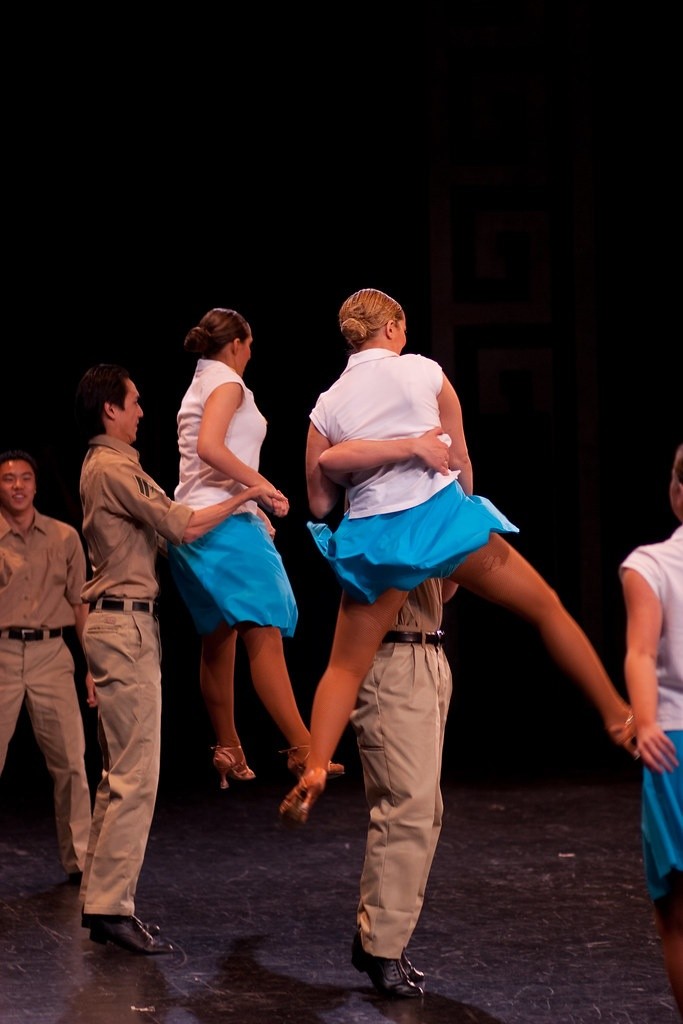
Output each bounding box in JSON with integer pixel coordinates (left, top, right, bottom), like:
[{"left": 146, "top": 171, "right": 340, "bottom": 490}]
[
  {"left": 278, "top": 746, "right": 345, "bottom": 781},
  {"left": 279, "top": 769, "right": 327, "bottom": 823},
  {"left": 211, "top": 745, "right": 256, "bottom": 790}
]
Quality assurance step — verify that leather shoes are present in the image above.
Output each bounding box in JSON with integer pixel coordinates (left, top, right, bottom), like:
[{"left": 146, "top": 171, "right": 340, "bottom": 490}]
[
  {"left": 81, "top": 905, "right": 160, "bottom": 934},
  {"left": 355, "top": 926, "right": 425, "bottom": 982},
  {"left": 90, "top": 915, "right": 174, "bottom": 954},
  {"left": 351, "top": 929, "right": 423, "bottom": 998}
]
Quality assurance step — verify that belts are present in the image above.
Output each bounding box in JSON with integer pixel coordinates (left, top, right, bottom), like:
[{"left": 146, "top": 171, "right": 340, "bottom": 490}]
[
  {"left": 90, "top": 599, "right": 158, "bottom": 614},
  {"left": 382, "top": 629, "right": 446, "bottom": 645},
  {"left": 0, "top": 629, "right": 62, "bottom": 641}
]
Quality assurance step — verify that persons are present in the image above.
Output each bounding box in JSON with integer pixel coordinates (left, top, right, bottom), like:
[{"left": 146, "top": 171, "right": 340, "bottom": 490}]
[
  {"left": 165, "top": 308, "right": 345, "bottom": 789},
  {"left": 80, "top": 362, "right": 285, "bottom": 955},
  {"left": 0, "top": 450, "right": 98, "bottom": 883},
  {"left": 619, "top": 446, "right": 683, "bottom": 1019},
  {"left": 279, "top": 289, "right": 638, "bottom": 824},
  {"left": 318, "top": 428, "right": 460, "bottom": 997}
]
